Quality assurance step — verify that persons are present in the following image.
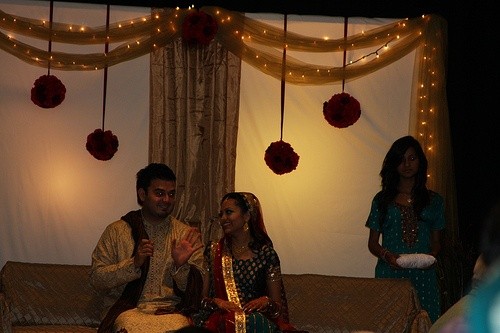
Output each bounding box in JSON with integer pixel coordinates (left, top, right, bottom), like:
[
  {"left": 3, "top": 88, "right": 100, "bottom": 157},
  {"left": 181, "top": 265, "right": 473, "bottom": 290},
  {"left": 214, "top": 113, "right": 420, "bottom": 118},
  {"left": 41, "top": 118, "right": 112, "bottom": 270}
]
[
  {"left": 366, "top": 135, "right": 445, "bottom": 323},
  {"left": 428, "top": 246, "right": 500, "bottom": 333},
  {"left": 88, "top": 163, "right": 206, "bottom": 333},
  {"left": 202, "top": 192, "right": 287, "bottom": 333}
]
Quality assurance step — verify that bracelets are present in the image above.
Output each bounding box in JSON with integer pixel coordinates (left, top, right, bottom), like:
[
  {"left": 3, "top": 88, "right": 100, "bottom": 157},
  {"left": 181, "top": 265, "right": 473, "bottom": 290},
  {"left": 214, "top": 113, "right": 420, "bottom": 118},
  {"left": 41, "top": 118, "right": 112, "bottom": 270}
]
[
  {"left": 382, "top": 251, "right": 390, "bottom": 259},
  {"left": 272, "top": 302, "right": 280, "bottom": 319},
  {"left": 373, "top": 244, "right": 381, "bottom": 255}
]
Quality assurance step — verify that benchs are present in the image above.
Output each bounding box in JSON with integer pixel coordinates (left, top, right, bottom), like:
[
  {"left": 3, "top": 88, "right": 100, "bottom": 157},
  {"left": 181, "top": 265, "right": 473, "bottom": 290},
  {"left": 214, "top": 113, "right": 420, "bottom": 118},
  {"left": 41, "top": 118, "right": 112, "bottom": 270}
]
[{"left": 0, "top": 261, "right": 433, "bottom": 333}]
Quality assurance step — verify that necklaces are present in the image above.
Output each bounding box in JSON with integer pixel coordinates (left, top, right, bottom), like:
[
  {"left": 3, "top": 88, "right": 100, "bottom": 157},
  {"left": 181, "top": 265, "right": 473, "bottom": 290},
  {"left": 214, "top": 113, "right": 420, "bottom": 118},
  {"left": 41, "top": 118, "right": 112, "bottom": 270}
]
[{"left": 407, "top": 199, "right": 411, "bottom": 203}]
